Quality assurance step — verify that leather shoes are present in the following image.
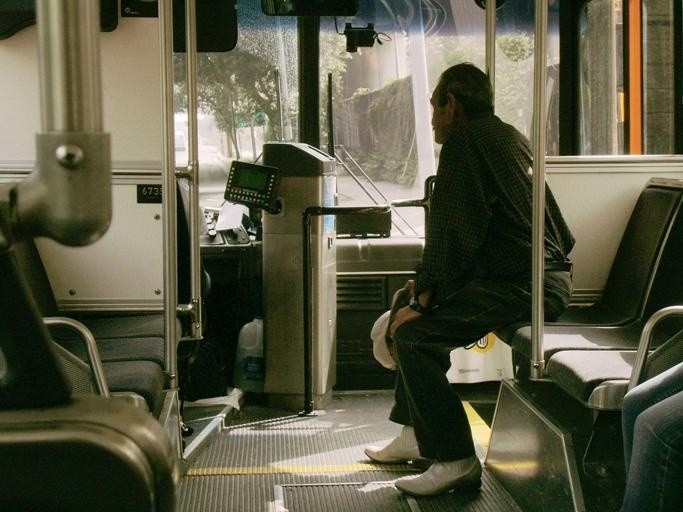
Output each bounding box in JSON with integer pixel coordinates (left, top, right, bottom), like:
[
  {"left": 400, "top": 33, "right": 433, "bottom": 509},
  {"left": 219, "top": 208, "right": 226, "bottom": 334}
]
[
  {"left": 365, "top": 436, "right": 432, "bottom": 469},
  {"left": 395, "top": 455, "right": 482, "bottom": 497}
]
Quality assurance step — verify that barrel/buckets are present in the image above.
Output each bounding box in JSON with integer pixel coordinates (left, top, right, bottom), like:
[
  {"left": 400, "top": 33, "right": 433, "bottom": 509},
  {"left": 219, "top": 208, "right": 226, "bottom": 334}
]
[{"left": 233, "top": 316, "right": 264, "bottom": 393}]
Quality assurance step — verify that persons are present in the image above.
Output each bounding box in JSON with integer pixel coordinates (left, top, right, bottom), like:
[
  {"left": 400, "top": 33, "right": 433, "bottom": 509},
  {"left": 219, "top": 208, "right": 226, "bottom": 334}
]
[
  {"left": 357, "top": 60, "right": 576, "bottom": 499},
  {"left": 174, "top": 175, "right": 212, "bottom": 304}
]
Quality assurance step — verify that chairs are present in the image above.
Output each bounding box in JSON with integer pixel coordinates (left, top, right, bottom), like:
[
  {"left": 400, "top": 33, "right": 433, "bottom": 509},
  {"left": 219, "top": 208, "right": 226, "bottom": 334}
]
[
  {"left": 511, "top": 178, "right": 681, "bottom": 476},
  {"left": 0, "top": 180, "right": 210, "bottom": 511}
]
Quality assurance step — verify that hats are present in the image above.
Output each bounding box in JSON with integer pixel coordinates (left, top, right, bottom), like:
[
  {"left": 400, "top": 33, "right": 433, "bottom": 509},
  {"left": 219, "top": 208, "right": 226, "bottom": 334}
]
[{"left": 370, "top": 288, "right": 410, "bottom": 370}]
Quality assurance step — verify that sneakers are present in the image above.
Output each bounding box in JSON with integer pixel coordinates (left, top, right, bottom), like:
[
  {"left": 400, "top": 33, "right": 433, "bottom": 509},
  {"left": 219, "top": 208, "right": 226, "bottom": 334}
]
[{"left": 179, "top": 416, "right": 194, "bottom": 436}]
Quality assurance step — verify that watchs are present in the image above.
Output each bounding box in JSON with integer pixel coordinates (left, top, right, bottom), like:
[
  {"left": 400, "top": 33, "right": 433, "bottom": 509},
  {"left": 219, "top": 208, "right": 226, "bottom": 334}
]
[{"left": 407, "top": 294, "right": 425, "bottom": 314}]
